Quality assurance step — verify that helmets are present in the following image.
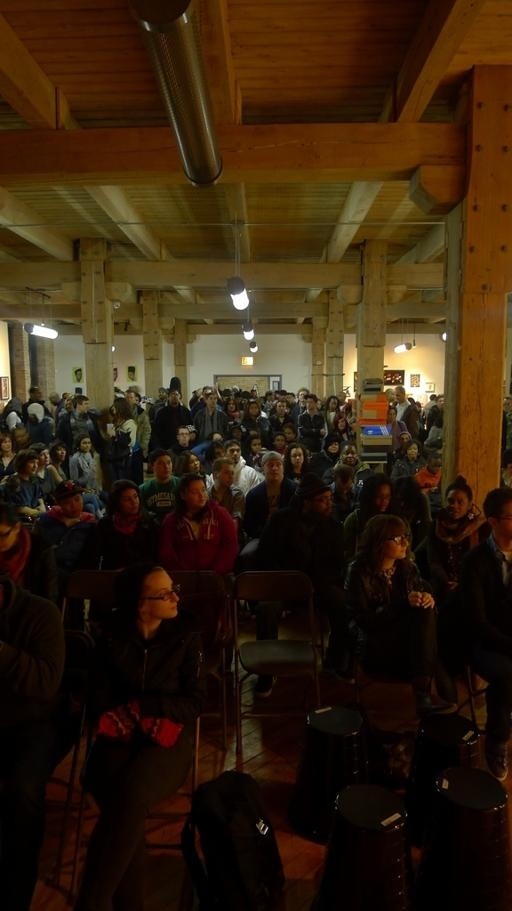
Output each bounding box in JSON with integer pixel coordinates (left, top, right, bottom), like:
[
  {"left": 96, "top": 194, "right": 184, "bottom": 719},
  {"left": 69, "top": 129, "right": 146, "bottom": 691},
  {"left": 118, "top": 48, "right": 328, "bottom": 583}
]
[{"left": 28, "top": 403, "right": 44, "bottom": 424}]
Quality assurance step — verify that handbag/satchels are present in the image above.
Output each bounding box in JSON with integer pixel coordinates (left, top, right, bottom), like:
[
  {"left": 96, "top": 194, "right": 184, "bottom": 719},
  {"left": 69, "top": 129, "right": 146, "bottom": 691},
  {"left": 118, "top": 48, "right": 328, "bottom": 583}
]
[
  {"left": 179, "top": 771, "right": 284, "bottom": 876},
  {"left": 100, "top": 440, "right": 129, "bottom": 463}
]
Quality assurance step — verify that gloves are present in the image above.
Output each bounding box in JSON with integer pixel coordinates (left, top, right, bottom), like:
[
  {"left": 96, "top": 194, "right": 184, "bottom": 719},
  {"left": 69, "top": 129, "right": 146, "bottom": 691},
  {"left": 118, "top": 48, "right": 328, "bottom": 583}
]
[
  {"left": 97, "top": 702, "right": 140, "bottom": 736},
  {"left": 142, "top": 717, "right": 182, "bottom": 745}
]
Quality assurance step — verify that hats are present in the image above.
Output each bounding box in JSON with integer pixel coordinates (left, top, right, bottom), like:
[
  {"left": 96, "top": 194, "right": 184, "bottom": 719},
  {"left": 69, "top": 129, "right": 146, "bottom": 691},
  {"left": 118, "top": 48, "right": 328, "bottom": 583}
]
[
  {"left": 295, "top": 473, "right": 331, "bottom": 497},
  {"left": 55, "top": 480, "right": 86, "bottom": 499}
]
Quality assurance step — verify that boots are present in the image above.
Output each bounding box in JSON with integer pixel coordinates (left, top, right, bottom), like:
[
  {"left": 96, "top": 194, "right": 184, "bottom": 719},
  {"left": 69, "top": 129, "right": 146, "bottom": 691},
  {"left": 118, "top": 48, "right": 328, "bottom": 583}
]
[{"left": 415, "top": 676, "right": 457, "bottom": 716}]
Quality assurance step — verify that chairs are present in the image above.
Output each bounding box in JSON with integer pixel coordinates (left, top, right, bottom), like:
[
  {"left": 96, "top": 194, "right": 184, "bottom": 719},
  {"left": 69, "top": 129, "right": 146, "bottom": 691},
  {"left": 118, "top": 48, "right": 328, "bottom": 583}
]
[{"left": 56, "top": 564, "right": 511, "bottom": 909}]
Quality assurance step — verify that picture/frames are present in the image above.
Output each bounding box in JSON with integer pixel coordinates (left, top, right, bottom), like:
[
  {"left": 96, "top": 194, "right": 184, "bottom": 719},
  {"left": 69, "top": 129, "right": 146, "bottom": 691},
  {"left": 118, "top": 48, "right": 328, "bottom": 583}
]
[{"left": 1, "top": 377, "right": 11, "bottom": 400}]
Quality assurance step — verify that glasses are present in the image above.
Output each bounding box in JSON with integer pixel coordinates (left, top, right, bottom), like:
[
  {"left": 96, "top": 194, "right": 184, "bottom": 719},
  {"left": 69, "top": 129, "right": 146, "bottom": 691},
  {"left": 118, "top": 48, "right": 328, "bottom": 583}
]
[
  {"left": 143, "top": 585, "right": 181, "bottom": 600},
  {"left": 388, "top": 532, "right": 410, "bottom": 542}
]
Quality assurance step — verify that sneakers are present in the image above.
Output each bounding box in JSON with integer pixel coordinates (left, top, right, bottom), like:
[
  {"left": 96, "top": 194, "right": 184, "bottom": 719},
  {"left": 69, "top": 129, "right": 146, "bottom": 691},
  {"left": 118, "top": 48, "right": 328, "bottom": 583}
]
[{"left": 484, "top": 733, "right": 508, "bottom": 781}]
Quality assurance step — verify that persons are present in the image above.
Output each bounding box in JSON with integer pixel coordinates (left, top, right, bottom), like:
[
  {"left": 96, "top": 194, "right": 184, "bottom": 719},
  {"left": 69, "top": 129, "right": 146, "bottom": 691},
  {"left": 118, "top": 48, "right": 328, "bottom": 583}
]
[
  {"left": 114, "top": 368, "right": 117, "bottom": 381},
  {"left": 1, "top": 384, "right": 512, "bottom": 909},
  {"left": 74, "top": 368, "right": 82, "bottom": 383},
  {"left": 128, "top": 366, "right": 135, "bottom": 381}
]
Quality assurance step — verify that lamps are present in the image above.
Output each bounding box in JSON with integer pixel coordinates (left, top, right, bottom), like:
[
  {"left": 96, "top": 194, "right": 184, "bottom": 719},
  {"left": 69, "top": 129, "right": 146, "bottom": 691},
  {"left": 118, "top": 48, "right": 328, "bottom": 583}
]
[
  {"left": 138, "top": 2, "right": 234, "bottom": 195},
  {"left": 227, "top": 217, "right": 260, "bottom": 354},
  {"left": 23, "top": 287, "right": 62, "bottom": 341},
  {"left": 393, "top": 310, "right": 415, "bottom": 354}
]
